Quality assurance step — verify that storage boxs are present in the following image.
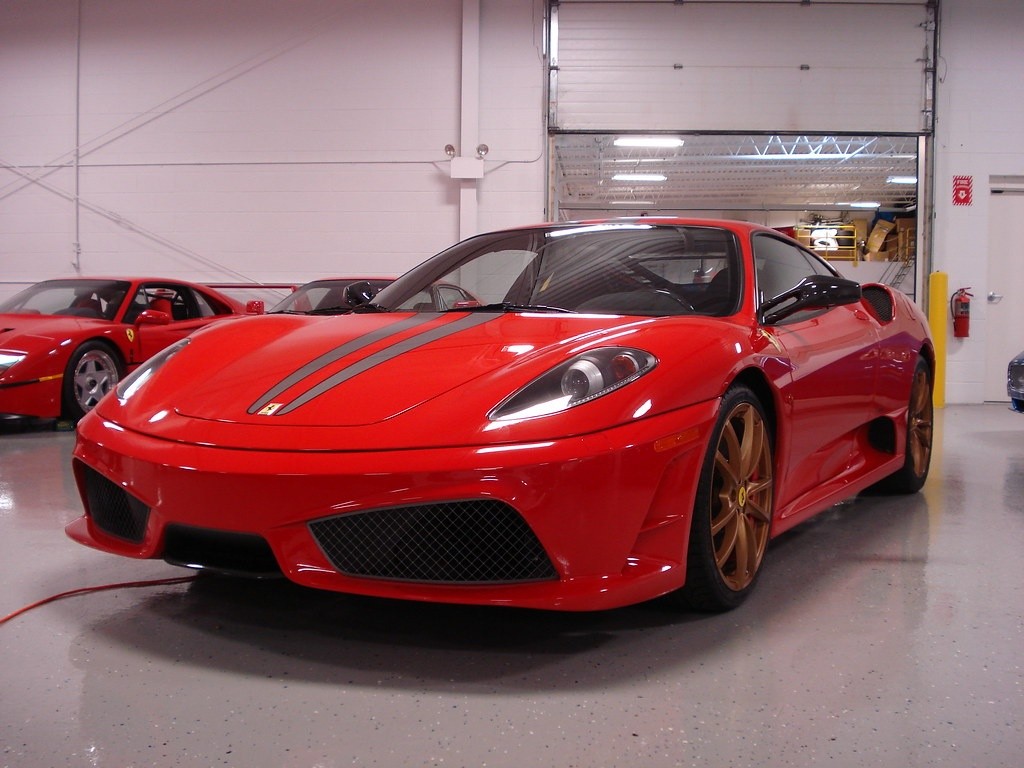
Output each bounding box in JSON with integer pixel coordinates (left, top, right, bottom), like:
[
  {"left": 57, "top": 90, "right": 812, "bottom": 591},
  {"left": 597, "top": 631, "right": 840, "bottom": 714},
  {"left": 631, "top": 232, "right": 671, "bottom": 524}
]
[{"left": 796, "top": 215, "right": 915, "bottom": 263}]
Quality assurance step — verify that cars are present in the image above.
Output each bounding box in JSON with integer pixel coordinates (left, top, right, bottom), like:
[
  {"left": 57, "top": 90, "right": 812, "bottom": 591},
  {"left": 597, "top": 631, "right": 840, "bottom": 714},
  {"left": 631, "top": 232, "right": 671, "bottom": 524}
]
[{"left": 266, "top": 274, "right": 485, "bottom": 314}]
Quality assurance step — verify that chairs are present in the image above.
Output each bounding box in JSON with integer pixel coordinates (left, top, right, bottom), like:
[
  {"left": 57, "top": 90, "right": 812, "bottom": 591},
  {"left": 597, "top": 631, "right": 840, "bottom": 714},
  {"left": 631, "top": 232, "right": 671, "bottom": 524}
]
[
  {"left": 76, "top": 297, "right": 102, "bottom": 315},
  {"left": 703, "top": 267, "right": 761, "bottom": 313}
]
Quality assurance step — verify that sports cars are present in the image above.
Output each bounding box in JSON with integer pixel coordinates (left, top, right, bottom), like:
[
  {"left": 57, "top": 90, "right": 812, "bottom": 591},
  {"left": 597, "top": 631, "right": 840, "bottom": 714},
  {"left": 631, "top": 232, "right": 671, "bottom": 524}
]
[
  {"left": 60, "top": 215, "right": 937, "bottom": 616},
  {"left": 0, "top": 274, "right": 313, "bottom": 429}
]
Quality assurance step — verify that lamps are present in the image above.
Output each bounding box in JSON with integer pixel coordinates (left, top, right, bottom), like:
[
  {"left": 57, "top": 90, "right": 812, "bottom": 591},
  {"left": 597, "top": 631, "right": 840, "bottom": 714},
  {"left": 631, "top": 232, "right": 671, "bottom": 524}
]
[{"left": 444, "top": 143, "right": 490, "bottom": 180}]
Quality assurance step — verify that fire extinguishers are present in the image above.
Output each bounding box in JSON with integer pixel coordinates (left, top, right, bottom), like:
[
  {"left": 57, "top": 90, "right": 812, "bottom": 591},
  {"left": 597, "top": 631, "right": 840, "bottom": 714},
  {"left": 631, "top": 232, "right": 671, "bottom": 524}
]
[{"left": 950, "top": 287, "right": 974, "bottom": 338}]
